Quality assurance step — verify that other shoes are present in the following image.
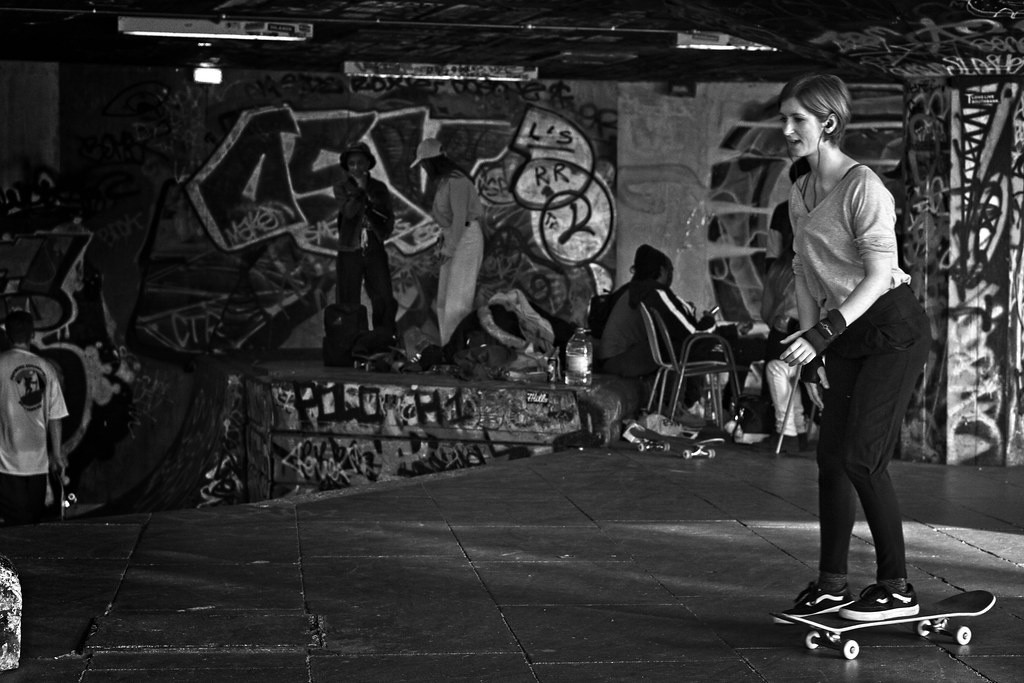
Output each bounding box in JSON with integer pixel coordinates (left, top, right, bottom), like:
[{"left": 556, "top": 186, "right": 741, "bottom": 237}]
[
  {"left": 753, "top": 434, "right": 807, "bottom": 453},
  {"left": 686, "top": 401, "right": 716, "bottom": 420}
]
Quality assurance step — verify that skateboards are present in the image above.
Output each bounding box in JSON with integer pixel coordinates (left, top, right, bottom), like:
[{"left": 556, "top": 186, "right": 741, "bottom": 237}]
[{"left": 769, "top": 589, "right": 998, "bottom": 661}]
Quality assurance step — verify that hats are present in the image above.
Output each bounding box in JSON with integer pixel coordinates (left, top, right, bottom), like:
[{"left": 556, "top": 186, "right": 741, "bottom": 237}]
[
  {"left": 409, "top": 137, "right": 445, "bottom": 168},
  {"left": 339, "top": 142, "right": 376, "bottom": 172}
]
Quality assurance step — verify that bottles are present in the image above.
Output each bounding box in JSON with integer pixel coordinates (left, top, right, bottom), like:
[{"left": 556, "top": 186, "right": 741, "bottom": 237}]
[{"left": 564, "top": 327, "right": 593, "bottom": 384}]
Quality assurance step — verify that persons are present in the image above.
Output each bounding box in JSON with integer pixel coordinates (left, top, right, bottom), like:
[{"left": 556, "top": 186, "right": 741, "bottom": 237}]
[
  {"left": 0, "top": 311, "right": 69, "bottom": 525},
  {"left": 778, "top": 73, "right": 932, "bottom": 622},
  {"left": 759, "top": 156, "right": 830, "bottom": 454},
  {"left": 590, "top": 246, "right": 728, "bottom": 447},
  {"left": 334, "top": 143, "right": 394, "bottom": 339},
  {"left": 410, "top": 138, "right": 485, "bottom": 346}
]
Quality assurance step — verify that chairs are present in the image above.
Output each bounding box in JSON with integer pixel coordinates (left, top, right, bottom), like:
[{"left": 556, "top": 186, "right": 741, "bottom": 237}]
[{"left": 638, "top": 300, "right": 752, "bottom": 433}]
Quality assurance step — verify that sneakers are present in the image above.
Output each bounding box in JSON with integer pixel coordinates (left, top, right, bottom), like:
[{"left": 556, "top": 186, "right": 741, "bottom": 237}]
[
  {"left": 773, "top": 582, "right": 854, "bottom": 625},
  {"left": 839, "top": 582, "right": 920, "bottom": 622}
]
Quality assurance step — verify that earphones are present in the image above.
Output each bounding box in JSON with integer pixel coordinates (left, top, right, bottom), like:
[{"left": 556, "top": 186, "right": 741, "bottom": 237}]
[{"left": 825, "top": 123, "right": 831, "bottom": 128}]
